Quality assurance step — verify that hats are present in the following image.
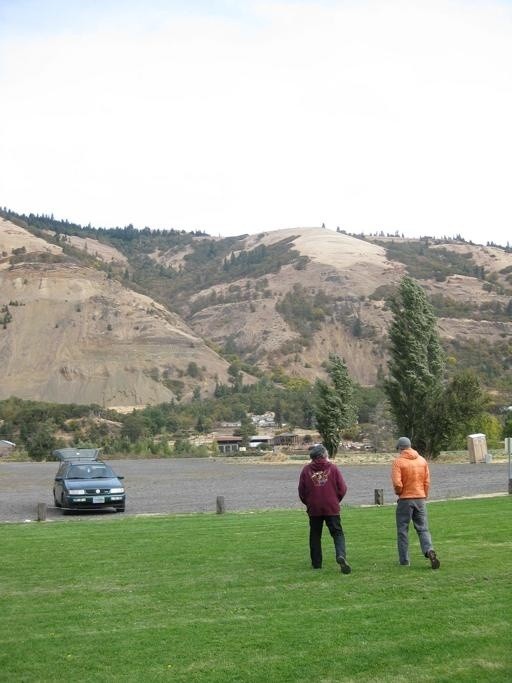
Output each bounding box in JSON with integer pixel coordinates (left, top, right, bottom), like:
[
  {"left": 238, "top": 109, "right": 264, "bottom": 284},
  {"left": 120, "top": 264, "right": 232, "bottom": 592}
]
[{"left": 396, "top": 436, "right": 412, "bottom": 450}]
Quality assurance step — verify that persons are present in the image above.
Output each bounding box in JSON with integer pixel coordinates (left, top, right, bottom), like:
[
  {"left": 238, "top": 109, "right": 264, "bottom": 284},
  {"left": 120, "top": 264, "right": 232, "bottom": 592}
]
[
  {"left": 298, "top": 444, "right": 351, "bottom": 575},
  {"left": 391, "top": 436, "right": 440, "bottom": 570}
]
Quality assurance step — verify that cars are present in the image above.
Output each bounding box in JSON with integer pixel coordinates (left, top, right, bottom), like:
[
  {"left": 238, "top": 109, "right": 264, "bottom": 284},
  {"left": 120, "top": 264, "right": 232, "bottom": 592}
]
[{"left": 51, "top": 448, "right": 126, "bottom": 516}]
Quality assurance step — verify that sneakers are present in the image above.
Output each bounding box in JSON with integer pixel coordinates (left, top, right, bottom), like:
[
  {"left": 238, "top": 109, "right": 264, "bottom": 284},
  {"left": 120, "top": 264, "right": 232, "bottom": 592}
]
[
  {"left": 336, "top": 555, "right": 352, "bottom": 575},
  {"left": 427, "top": 548, "right": 441, "bottom": 569}
]
